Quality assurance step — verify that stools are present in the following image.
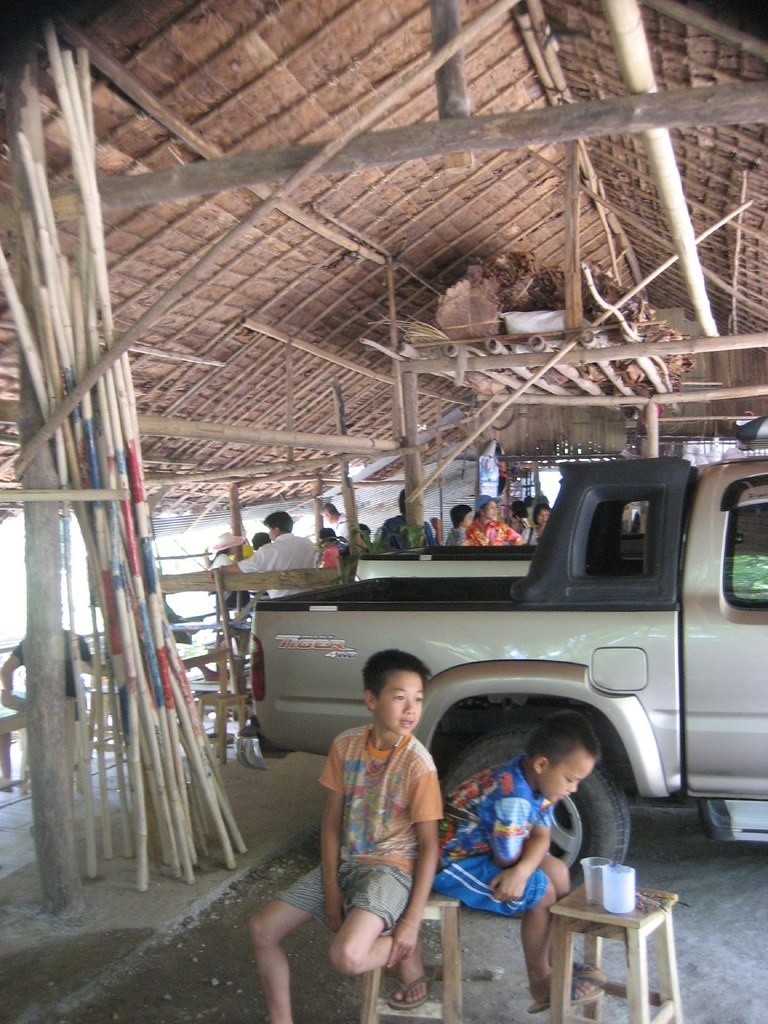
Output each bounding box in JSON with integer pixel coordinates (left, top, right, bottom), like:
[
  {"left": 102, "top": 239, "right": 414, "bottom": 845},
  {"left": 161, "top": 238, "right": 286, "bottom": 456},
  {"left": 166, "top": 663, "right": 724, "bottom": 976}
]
[
  {"left": 360, "top": 890, "right": 464, "bottom": 1024},
  {"left": 549, "top": 880, "right": 684, "bottom": 1024},
  {"left": 90, "top": 686, "right": 124, "bottom": 762},
  {"left": 197, "top": 693, "right": 248, "bottom": 764}
]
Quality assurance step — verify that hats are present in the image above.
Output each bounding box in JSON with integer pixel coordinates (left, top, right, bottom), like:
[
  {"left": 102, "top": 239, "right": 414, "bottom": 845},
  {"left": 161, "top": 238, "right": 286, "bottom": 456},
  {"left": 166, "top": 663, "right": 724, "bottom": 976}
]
[
  {"left": 212, "top": 532, "right": 246, "bottom": 551},
  {"left": 475, "top": 495, "right": 502, "bottom": 515}
]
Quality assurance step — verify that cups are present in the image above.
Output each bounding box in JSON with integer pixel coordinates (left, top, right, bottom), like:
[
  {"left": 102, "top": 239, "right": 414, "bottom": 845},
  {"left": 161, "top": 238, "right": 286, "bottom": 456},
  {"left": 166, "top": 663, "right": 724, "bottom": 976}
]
[
  {"left": 602, "top": 863, "right": 636, "bottom": 914},
  {"left": 580, "top": 856, "right": 613, "bottom": 906}
]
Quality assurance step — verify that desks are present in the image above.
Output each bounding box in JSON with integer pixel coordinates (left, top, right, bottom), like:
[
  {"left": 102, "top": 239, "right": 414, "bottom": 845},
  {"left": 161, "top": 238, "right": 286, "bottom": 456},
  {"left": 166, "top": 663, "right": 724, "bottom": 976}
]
[
  {"left": 1, "top": 689, "right": 77, "bottom": 797},
  {"left": 91, "top": 644, "right": 231, "bottom": 758}
]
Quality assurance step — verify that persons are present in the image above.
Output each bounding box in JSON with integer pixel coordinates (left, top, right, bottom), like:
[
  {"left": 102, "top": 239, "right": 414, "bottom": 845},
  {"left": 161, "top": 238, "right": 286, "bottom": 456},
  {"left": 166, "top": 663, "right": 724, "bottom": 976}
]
[
  {"left": 0, "top": 602, "right": 94, "bottom": 838},
  {"left": 431, "top": 712, "right": 603, "bottom": 1014},
  {"left": 207, "top": 489, "right": 552, "bottom": 611},
  {"left": 250, "top": 648, "right": 444, "bottom": 1024}
]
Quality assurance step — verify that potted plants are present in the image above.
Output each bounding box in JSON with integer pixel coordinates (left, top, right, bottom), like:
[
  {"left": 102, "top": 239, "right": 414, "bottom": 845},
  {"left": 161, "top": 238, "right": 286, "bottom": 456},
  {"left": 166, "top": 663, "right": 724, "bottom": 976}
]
[{"left": 314, "top": 522, "right": 371, "bottom": 583}]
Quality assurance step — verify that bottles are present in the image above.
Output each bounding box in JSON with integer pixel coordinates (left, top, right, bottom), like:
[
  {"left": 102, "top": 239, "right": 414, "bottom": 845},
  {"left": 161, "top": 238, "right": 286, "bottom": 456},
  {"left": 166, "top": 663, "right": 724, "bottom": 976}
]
[
  {"left": 607, "top": 861, "right": 621, "bottom": 873},
  {"left": 551, "top": 440, "right": 604, "bottom": 455}
]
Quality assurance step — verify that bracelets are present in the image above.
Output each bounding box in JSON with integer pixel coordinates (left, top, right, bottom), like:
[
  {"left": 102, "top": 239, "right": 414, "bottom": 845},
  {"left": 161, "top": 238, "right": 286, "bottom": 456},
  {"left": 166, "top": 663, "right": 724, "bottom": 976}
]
[{"left": 400, "top": 916, "right": 423, "bottom": 932}]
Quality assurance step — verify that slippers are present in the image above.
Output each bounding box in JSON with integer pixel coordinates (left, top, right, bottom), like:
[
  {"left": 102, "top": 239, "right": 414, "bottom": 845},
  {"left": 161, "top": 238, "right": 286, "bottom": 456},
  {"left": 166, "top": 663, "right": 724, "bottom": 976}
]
[
  {"left": 386, "top": 964, "right": 438, "bottom": 1012},
  {"left": 573, "top": 961, "right": 605, "bottom": 984},
  {"left": 527, "top": 977, "right": 605, "bottom": 1014}
]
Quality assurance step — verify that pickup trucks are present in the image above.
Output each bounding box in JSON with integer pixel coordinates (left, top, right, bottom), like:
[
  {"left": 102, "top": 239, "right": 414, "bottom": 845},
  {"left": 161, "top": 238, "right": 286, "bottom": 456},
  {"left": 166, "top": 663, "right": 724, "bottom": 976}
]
[{"left": 237, "top": 416, "right": 768, "bottom": 919}]
